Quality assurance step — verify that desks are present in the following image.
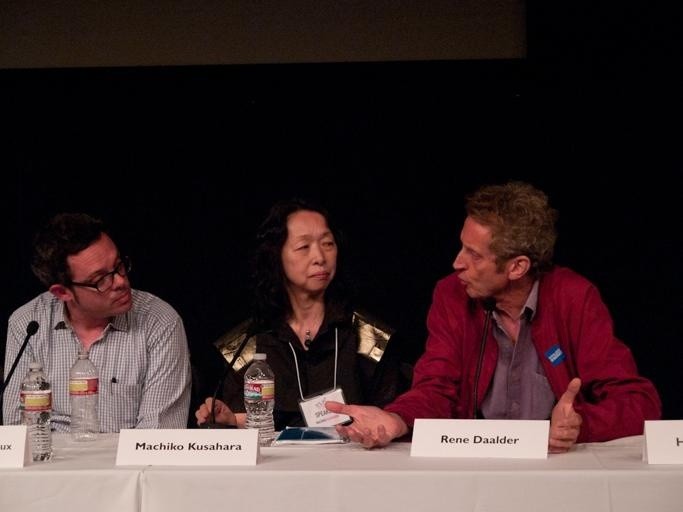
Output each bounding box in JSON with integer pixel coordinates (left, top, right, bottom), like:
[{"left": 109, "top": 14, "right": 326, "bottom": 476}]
[{"left": 1, "top": 425, "right": 682, "bottom": 510}]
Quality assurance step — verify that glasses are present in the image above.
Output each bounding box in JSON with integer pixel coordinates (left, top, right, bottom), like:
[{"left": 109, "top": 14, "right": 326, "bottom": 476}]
[{"left": 69, "top": 255, "right": 133, "bottom": 295}]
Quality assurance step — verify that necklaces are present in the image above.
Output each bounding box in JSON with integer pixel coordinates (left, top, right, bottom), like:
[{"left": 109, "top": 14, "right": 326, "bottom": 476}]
[{"left": 292, "top": 310, "right": 323, "bottom": 339}]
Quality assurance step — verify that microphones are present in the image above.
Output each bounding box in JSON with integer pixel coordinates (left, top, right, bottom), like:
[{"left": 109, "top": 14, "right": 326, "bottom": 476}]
[
  {"left": 201, "top": 321, "right": 263, "bottom": 428},
  {"left": 0, "top": 321, "right": 39, "bottom": 395},
  {"left": 473, "top": 296, "right": 496, "bottom": 419}
]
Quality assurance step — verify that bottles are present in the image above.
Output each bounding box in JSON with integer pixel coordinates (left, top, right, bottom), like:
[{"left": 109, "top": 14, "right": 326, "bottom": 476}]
[
  {"left": 243, "top": 351, "right": 276, "bottom": 448},
  {"left": 68, "top": 350, "right": 101, "bottom": 441},
  {"left": 18, "top": 362, "right": 52, "bottom": 462}
]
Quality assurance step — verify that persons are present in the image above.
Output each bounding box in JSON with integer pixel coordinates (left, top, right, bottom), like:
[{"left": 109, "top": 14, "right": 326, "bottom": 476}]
[
  {"left": 194, "top": 202, "right": 411, "bottom": 430},
  {"left": 3, "top": 214, "right": 193, "bottom": 433},
  {"left": 323, "top": 179, "right": 665, "bottom": 454}
]
